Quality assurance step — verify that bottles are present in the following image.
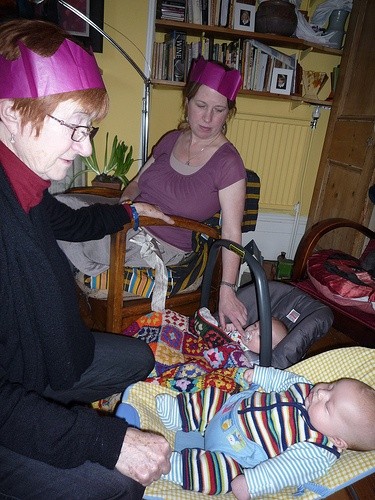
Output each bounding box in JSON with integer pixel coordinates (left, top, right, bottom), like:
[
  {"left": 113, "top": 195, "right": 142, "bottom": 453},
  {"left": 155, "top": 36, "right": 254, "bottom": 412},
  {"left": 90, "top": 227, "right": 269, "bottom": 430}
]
[
  {"left": 254, "top": 0, "right": 298, "bottom": 36},
  {"left": 277, "top": 252, "right": 286, "bottom": 264}
]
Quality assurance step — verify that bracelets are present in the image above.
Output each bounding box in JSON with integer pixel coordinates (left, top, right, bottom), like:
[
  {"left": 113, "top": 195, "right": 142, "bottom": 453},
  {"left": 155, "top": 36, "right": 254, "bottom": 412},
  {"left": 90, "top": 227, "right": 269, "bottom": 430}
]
[
  {"left": 122, "top": 200, "right": 132, "bottom": 204},
  {"left": 131, "top": 204, "right": 139, "bottom": 231}
]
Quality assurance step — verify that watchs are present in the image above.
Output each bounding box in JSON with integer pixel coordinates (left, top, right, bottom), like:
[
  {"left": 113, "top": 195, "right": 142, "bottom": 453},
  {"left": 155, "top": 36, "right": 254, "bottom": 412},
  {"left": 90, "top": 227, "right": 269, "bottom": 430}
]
[{"left": 220, "top": 282, "right": 238, "bottom": 292}]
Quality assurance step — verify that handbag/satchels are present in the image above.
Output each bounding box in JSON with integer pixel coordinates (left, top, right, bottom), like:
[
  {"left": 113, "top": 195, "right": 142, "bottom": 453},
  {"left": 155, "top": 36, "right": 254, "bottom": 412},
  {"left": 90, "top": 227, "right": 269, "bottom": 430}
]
[{"left": 360, "top": 250, "right": 375, "bottom": 272}]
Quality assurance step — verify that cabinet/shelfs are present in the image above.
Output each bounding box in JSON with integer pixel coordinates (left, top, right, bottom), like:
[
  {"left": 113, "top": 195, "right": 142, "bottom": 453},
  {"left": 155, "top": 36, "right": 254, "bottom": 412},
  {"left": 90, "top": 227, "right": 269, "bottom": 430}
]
[{"left": 149, "top": 0, "right": 369, "bottom": 108}]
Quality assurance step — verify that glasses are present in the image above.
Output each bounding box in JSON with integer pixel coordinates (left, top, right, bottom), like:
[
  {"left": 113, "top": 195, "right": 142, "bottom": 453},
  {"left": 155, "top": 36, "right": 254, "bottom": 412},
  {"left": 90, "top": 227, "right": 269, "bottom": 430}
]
[{"left": 46, "top": 114, "right": 99, "bottom": 142}]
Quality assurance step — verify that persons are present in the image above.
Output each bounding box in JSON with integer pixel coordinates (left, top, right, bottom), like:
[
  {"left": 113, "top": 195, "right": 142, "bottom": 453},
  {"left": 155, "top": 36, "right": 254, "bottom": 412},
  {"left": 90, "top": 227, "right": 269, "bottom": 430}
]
[
  {"left": 123, "top": 318, "right": 288, "bottom": 379},
  {"left": 276, "top": 75, "right": 286, "bottom": 89},
  {"left": 54, "top": 58, "right": 248, "bottom": 337},
  {"left": 155, "top": 364, "right": 375, "bottom": 500},
  {"left": 240, "top": 11, "right": 249, "bottom": 25},
  {"left": 1, "top": 18, "right": 172, "bottom": 499}
]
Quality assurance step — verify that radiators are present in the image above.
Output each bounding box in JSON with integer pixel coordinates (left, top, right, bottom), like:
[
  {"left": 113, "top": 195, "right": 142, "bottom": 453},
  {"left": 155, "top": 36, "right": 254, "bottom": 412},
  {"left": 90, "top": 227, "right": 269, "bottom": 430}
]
[{"left": 226, "top": 109, "right": 318, "bottom": 211}]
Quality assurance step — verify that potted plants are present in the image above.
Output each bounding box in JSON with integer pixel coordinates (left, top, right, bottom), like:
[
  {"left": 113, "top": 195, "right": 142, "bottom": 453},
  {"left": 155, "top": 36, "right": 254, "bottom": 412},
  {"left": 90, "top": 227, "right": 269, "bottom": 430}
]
[{"left": 69, "top": 132, "right": 134, "bottom": 189}]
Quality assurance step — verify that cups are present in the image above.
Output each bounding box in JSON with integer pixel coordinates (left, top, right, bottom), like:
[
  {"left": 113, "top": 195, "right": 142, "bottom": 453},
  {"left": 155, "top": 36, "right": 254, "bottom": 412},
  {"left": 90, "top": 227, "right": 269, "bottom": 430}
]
[{"left": 325, "top": 9, "right": 349, "bottom": 50}]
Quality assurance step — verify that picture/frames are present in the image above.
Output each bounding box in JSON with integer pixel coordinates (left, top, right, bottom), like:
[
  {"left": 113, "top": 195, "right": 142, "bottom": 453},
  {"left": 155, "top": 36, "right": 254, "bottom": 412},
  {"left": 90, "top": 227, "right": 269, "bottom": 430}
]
[
  {"left": 0, "top": 0, "right": 104, "bottom": 54},
  {"left": 269, "top": 67, "right": 294, "bottom": 96},
  {"left": 234, "top": 4, "right": 256, "bottom": 32}
]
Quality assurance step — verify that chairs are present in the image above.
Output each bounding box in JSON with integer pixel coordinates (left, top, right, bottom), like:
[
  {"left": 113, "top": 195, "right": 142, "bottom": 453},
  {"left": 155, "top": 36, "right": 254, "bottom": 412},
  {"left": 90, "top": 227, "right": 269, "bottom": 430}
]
[
  {"left": 90, "top": 279, "right": 334, "bottom": 412},
  {"left": 114, "top": 346, "right": 375, "bottom": 500},
  {"left": 283, "top": 184, "right": 375, "bottom": 349},
  {"left": 72, "top": 168, "right": 261, "bottom": 334}
]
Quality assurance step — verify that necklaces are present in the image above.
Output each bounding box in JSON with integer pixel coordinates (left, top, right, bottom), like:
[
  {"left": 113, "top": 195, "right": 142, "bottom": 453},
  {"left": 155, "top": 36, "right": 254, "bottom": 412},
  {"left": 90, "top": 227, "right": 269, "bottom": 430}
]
[{"left": 185, "top": 142, "right": 210, "bottom": 168}]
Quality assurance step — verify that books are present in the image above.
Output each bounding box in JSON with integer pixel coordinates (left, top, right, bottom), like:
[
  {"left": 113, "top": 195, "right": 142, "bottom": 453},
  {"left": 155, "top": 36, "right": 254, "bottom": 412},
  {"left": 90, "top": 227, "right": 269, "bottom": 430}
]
[
  {"left": 154, "top": 32, "right": 275, "bottom": 92},
  {"left": 160, "top": 0, "right": 236, "bottom": 29}
]
